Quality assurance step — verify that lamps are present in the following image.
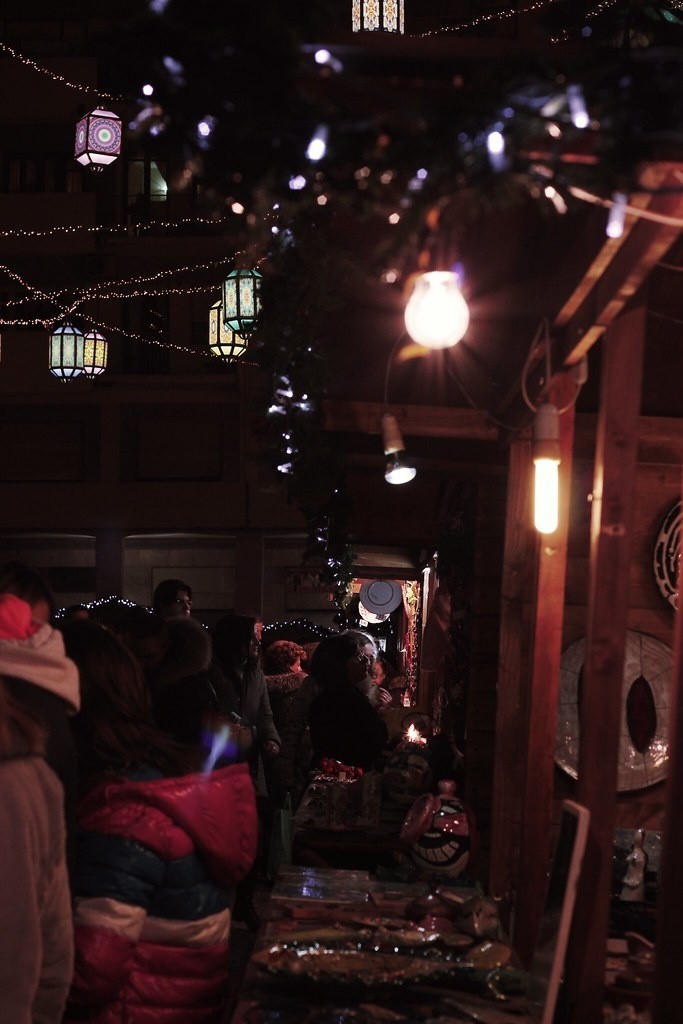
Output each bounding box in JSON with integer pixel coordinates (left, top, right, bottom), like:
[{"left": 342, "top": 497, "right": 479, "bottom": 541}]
[
  {"left": 532, "top": 403, "right": 562, "bottom": 535},
  {"left": 82, "top": 326, "right": 109, "bottom": 383},
  {"left": 208, "top": 298, "right": 250, "bottom": 366},
  {"left": 378, "top": 413, "right": 418, "bottom": 486},
  {"left": 48, "top": 317, "right": 85, "bottom": 384},
  {"left": 402, "top": 269, "right": 473, "bottom": 350},
  {"left": 72, "top": 97, "right": 125, "bottom": 177},
  {"left": 221, "top": 265, "right": 263, "bottom": 342}
]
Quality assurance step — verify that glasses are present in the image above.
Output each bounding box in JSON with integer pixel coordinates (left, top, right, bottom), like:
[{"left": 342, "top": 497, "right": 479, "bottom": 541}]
[
  {"left": 347, "top": 649, "right": 365, "bottom": 664},
  {"left": 163, "top": 598, "right": 190, "bottom": 608}
]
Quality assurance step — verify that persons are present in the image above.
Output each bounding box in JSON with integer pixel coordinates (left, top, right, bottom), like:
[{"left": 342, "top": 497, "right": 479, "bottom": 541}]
[
  {"left": 58, "top": 637, "right": 261, "bottom": 1024},
  {"left": 0, "top": 587, "right": 80, "bottom": 1024},
  {"left": 1, "top": 561, "right": 408, "bottom": 903}
]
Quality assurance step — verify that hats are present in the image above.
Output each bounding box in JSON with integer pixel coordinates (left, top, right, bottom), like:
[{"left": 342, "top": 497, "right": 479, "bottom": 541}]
[{"left": 0, "top": 590, "right": 83, "bottom": 716}]
